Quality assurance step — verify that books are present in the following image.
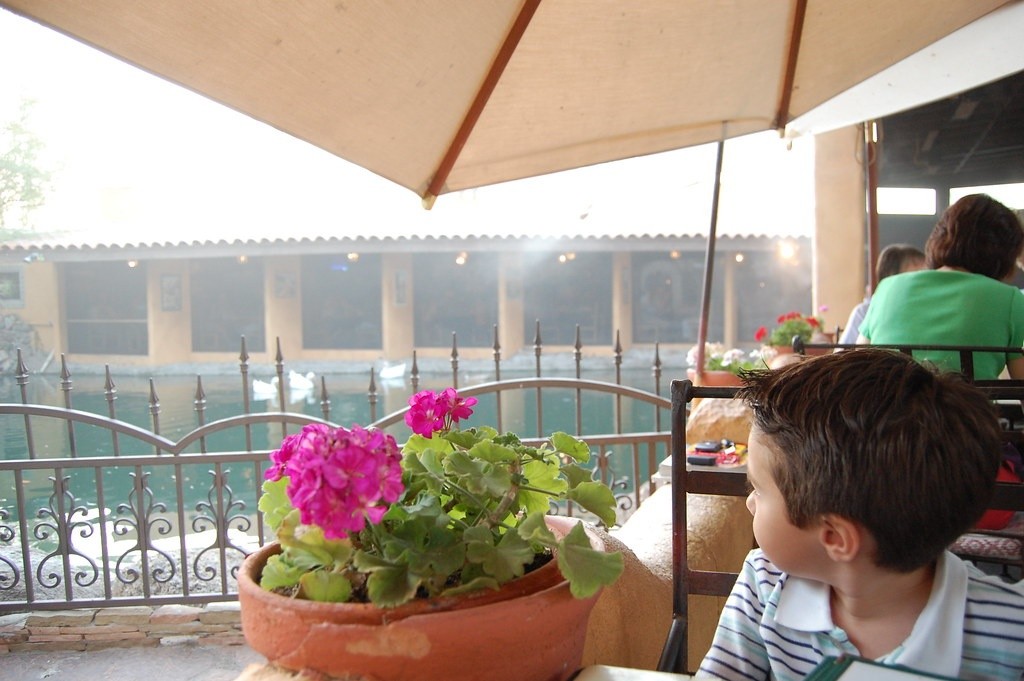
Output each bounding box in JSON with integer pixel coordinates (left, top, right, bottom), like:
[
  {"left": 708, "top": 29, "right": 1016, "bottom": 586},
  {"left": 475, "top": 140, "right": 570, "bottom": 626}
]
[{"left": 651, "top": 441, "right": 749, "bottom": 484}]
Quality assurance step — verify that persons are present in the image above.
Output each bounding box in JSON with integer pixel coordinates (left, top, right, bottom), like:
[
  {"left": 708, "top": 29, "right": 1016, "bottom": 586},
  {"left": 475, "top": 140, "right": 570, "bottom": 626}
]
[
  {"left": 832, "top": 245, "right": 925, "bottom": 355},
  {"left": 689, "top": 349, "right": 1024, "bottom": 681},
  {"left": 856, "top": 193, "right": 1024, "bottom": 402}
]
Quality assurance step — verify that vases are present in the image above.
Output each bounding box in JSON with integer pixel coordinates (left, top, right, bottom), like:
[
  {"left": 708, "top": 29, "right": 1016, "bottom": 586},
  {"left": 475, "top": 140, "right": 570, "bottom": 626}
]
[
  {"left": 687, "top": 369, "right": 757, "bottom": 388},
  {"left": 775, "top": 332, "right": 833, "bottom": 357},
  {"left": 237, "top": 513, "right": 605, "bottom": 681}
]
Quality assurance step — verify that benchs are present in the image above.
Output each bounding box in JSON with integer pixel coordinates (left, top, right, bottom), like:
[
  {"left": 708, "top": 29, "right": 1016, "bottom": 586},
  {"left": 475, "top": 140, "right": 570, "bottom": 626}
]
[{"left": 656, "top": 344, "right": 1024, "bottom": 681}]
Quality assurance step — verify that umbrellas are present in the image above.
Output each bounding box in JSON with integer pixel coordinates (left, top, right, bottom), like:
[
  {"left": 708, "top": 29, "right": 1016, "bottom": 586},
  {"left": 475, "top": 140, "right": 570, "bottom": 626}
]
[{"left": 0, "top": 0, "right": 1011, "bottom": 210}]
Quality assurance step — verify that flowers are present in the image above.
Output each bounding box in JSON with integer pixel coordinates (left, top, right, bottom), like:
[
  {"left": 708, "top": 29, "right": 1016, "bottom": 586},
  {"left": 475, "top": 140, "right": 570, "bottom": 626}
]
[
  {"left": 755, "top": 306, "right": 828, "bottom": 343},
  {"left": 686, "top": 341, "right": 779, "bottom": 373},
  {"left": 260, "top": 386, "right": 623, "bottom": 608}
]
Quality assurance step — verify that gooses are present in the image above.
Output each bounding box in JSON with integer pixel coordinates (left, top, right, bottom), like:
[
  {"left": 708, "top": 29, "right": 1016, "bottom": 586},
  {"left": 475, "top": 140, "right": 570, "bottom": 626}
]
[
  {"left": 382, "top": 378, "right": 405, "bottom": 388},
  {"left": 253, "top": 388, "right": 316, "bottom": 407},
  {"left": 253, "top": 362, "right": 406, "bottom": 393}
]
